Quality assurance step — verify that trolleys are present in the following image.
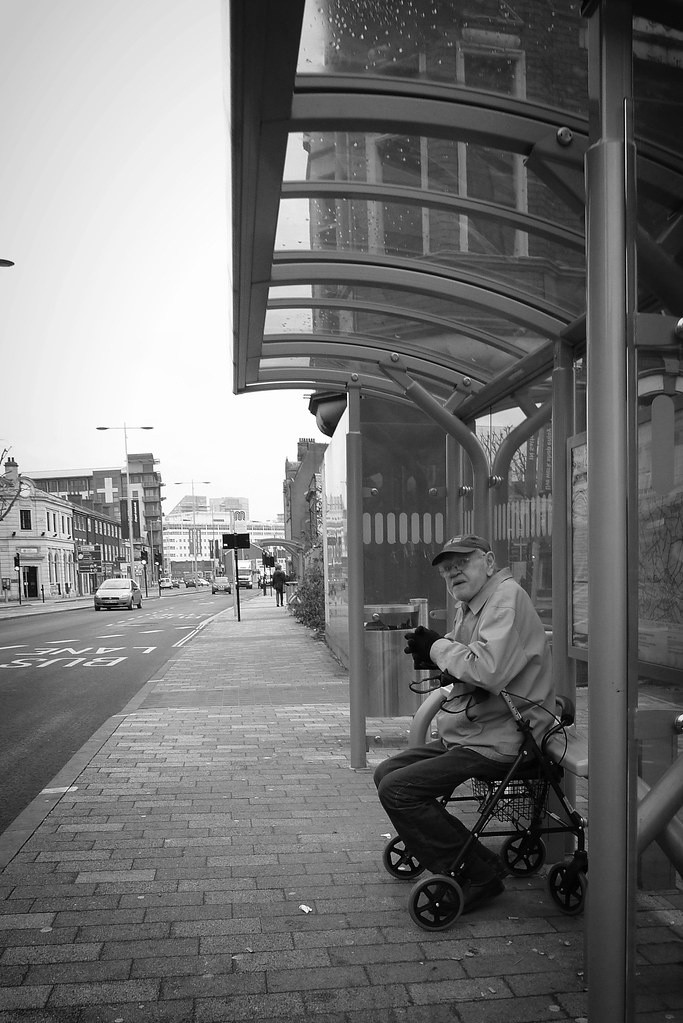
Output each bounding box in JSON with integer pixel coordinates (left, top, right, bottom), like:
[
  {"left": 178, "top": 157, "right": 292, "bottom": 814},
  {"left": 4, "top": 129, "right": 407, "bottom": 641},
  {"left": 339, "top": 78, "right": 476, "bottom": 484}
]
[{"left": 382, "top": 685, "right": 592, "bottom": 936}]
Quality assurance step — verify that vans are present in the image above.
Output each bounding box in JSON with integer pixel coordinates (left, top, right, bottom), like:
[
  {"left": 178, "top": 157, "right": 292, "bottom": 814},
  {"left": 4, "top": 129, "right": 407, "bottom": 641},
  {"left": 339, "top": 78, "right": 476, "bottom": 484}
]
[{"left": 160, "top": 578, "right": 173, "bottom": 590}]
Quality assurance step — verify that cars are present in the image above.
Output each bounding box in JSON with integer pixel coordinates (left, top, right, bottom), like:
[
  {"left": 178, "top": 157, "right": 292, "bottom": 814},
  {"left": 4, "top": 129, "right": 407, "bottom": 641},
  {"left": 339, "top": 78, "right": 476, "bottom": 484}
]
[
  {"left": 186, "top": 579, "right": 196, "bottom": 588},
  {"left": 172, "top": 580, "right": 179, "bottom": 588},
  {"left": 196, "top": 579, "right": 210, "bottom": 586},
  {"left": 93, "top": 578, "right": 143, "bottom": 611}
]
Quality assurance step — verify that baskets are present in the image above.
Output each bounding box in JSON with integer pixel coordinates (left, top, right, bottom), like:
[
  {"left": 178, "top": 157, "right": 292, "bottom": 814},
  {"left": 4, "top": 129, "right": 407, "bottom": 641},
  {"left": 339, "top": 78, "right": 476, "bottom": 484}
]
[{"left": 471, "top": 778, "right": 549, "bottom": 822}]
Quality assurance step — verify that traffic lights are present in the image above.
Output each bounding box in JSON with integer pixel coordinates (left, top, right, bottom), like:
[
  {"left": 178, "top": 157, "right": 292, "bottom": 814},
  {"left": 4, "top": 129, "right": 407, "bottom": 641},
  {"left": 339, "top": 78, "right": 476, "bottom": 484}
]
[
  {"left": 13, "top": 556, "right": 19, "bottom": 568},
  {"left": 140, "top": 551, "right": 148, "bottom": 565},
  {"left": 154, "top": 553, "right": 161, "bottom": 566},
  {"left": 262, "top": 552, "right": 266, "bottom": 566}
]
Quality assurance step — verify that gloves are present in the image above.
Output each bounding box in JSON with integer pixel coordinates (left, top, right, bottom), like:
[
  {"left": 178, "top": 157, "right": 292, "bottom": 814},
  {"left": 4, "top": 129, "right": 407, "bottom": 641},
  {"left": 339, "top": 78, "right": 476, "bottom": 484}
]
[{"left": 404, "top": 626, "right": 442, "bottom": 662}]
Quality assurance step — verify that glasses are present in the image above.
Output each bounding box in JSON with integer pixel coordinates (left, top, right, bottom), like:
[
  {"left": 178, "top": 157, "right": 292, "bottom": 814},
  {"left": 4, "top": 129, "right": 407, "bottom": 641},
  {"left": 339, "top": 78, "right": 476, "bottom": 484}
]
[{"left": 438, "top": 555, "right": 485, "bottom": 575}]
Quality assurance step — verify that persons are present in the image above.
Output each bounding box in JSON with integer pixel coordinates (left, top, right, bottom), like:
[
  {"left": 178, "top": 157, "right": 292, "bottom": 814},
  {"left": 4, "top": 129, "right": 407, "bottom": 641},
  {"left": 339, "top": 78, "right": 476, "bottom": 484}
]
[
  {"left": 272, "top": 564, "right": 292, "bottom": 607},
  {"left": 373, "top": 535, "right": 555, "bottom": 917}
]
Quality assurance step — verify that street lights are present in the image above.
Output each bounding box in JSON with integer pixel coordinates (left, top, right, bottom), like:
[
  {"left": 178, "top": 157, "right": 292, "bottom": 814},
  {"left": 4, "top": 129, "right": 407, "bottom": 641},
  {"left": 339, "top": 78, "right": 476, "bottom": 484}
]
[
  {"left": 95, "top": 423, "right": 154, "bottom": 581},
  {"left": 174, "top": 480, "right": 212, "bottom": 577}
]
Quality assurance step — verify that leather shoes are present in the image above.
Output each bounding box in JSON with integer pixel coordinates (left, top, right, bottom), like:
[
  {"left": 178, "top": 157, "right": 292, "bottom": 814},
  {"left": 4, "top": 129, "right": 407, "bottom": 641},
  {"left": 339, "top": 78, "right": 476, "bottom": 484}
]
[
  {"left": 426, "top": 875, "right": 504, "bottom": 914},
  {"left": 485, "top": 855, "right": 512, "bottom": 880}
]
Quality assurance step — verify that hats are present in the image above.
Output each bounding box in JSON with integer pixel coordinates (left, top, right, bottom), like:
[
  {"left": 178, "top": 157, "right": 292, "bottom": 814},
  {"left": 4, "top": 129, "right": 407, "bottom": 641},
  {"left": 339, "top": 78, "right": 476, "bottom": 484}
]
[{"left": 432, "top": 535, "right": 491, "bottom": 565}]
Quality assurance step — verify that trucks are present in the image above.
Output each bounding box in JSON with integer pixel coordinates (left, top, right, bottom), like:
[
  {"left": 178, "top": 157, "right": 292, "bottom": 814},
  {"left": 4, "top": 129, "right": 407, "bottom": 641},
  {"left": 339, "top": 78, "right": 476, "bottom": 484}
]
[
  {"left": 235, "top": 559, "right": 253, "bottom": 590},
  {"left": 258, "top": 565, "right": 275, "bottom": 589}
]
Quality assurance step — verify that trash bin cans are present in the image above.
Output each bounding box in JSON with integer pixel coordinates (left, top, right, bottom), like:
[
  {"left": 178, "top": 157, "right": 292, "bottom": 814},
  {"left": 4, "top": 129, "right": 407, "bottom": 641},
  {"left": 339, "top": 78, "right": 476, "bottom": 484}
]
[
  {"left": 285, "top": 582, "right": 298, "bottom": 605},
  {"left": 363, "top": 603, "right": 421, "bottom": 718}
]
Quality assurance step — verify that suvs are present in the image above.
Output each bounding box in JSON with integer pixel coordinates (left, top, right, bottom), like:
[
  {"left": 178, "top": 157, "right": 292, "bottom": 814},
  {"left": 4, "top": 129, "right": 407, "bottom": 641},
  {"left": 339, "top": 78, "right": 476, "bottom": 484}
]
[{"left": 212, "top": 577, "right": 231, "bottom": 595}]
